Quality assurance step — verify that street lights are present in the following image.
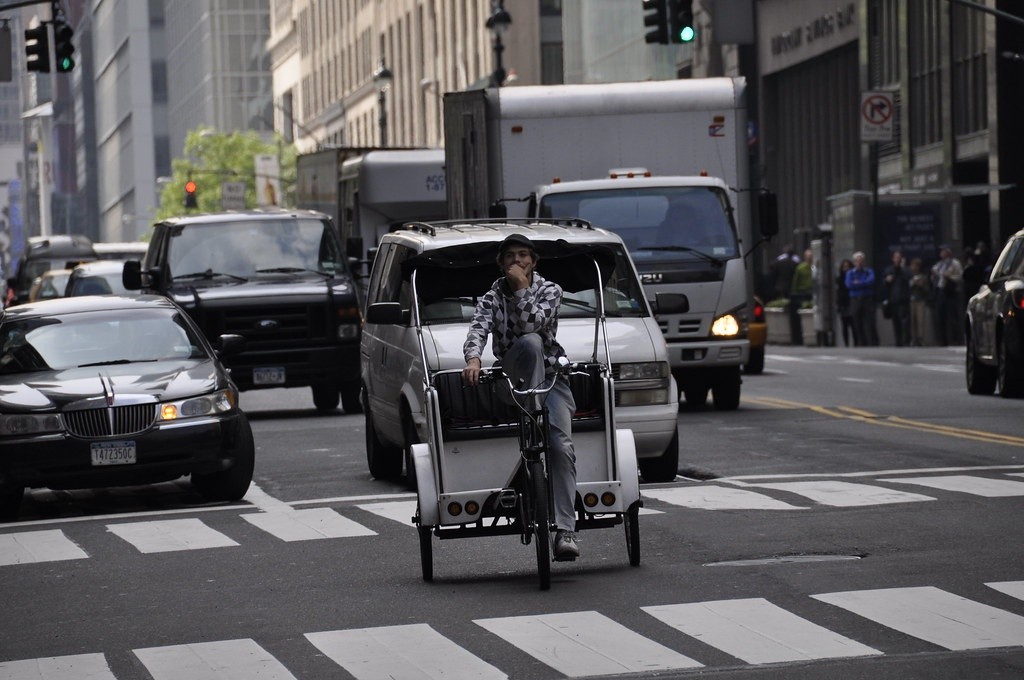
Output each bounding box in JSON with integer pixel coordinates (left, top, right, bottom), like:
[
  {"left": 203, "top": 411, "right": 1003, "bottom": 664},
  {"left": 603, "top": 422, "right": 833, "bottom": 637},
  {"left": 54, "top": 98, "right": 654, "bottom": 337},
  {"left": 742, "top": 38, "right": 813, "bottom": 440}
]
[
  {"left": 486, "top": 0, "right": 515, "bottom": 91},
  {"left": 375, "top": 55, "right": 395, "bottom": 147}
]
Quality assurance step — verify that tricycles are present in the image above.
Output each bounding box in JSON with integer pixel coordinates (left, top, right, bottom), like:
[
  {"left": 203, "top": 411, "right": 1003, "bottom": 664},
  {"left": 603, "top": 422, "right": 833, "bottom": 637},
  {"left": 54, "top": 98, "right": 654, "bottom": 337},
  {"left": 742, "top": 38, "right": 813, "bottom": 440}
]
[{"left": 395, "top": 236, "right": 644, "bottom": 591}]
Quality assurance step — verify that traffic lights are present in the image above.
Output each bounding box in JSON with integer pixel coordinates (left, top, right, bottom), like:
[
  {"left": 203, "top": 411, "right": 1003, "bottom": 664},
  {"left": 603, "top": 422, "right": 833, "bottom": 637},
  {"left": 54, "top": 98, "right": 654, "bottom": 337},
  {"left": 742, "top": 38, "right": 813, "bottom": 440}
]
[
  {"left": 640, "top": 0, "right": 668, "bottom": 45},
  {"left": 185, "top": 183, "right": 199, "bottom": 212},
  {"left": 669, "top": 0, "right": 698, "bottom": 46},
  {"left": 24, "top": 24, "right": 51, "bottom": 73},
  {"left": 52, "top": 19, "right": 76, "bottom": 72}
]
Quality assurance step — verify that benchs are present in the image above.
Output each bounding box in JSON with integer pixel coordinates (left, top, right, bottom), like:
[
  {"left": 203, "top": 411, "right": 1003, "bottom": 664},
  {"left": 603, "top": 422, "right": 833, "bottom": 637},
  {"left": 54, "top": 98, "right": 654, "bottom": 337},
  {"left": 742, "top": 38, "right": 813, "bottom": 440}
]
[{"left": 432, "top": 360, "right": 606, "bottom": 443}]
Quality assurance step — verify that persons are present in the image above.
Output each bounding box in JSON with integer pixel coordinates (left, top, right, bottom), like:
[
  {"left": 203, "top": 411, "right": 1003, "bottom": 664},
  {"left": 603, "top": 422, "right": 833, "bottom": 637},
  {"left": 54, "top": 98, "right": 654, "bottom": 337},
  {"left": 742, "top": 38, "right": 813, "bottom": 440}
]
[
  {"left": 884, "top": 249, "right": 913, "bottom": 346},
  {"left": 844, "top": 256, "right": 875, "bottom": 345},
  {"left": 461, "top": 234, "right": 580, "bottom": 558},
  {"left": 908, "top": 257, "right": 932, "bottom": 346},
  {"left": 932, "top": 242, "right": 992, "bottom": 346},
  {"left": 772, "top": 243, "right": 813, "bottom": 295},
  {"left": 837, "top": 262, "right": 857, "bottom": 347}
]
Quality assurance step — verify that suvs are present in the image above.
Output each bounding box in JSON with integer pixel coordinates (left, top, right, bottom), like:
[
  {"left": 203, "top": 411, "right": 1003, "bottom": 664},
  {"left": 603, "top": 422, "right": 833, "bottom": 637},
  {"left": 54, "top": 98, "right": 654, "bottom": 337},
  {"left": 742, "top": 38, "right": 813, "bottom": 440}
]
[{"left": 120, "top": 208, "right": 365, "bottom": 418}]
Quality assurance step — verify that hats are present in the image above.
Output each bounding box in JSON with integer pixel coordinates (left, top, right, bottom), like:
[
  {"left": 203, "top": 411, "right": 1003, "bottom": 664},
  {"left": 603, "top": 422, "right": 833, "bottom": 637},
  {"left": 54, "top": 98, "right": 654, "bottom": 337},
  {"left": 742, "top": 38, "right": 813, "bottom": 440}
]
[
  {"left": 498, "top": 233, "right": 534, "bottom": 254},
  {"left": 939, "top": 243, "right": 954, "bottom": 251}
]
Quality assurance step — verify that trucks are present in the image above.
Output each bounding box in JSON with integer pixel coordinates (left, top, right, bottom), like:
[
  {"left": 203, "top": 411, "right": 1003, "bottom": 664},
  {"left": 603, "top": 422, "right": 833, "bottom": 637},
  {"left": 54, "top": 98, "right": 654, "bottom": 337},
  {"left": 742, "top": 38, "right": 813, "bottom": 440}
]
[
  {"left": 291, "top": 144, "right": 448, "bottom": 307},
  {"left": 440, "top": 73, "right": 780, "bottom": 414}
]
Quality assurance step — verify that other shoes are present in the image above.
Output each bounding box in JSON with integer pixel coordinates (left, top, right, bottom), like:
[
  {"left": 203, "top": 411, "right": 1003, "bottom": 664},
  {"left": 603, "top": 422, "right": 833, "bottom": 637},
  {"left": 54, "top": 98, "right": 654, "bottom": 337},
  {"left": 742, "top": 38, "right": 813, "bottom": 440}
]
[
  {"left": 522, "top": 410, "right": 548, "bottom": 428},
  {"left": 553, "top": 530, "right": 581, "bottom": 557}
]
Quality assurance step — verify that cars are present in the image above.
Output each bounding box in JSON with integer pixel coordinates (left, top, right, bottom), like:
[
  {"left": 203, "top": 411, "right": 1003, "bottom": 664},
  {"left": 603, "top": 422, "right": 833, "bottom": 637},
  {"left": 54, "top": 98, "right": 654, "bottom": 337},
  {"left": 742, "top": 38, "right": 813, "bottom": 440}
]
[
  {"left": 0, "top": 234, "right": 153, "bottom": 308},
  {"left": 958, "top": 229, "right": 1024, "bottom": 398},
  {"left": 2, "top": 294, "right": 258, "bottom": 510}
]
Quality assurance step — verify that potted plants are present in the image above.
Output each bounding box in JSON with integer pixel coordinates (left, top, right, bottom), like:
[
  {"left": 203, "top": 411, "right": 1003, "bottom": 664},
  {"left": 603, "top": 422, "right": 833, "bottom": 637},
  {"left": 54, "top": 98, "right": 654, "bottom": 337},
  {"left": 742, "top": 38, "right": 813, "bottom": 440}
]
[
  {"left": 797, "top": 299, "right": 817, "bottom": 346},
  {"left": 764, "top": 299, "right": 798, "bottom": 343}
]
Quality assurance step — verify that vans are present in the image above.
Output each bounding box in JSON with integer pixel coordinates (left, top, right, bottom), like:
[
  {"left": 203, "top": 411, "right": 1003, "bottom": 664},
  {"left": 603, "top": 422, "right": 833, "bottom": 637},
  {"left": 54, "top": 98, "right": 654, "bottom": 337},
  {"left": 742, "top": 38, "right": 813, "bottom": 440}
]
[{"left": 360, "top": 217, "right": 682, "bottom": 491}]
